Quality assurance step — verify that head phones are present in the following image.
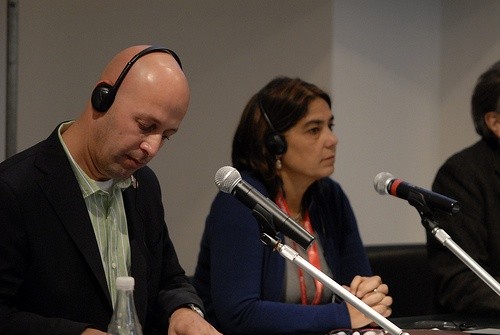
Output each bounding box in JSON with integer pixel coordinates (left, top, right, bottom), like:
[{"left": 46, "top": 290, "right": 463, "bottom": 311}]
[
  {"left": 91, "top": 46, "right": 183, "bottom": 112},
  {"left": 255, "top": 93, "right": 288, "bottom": 156}
]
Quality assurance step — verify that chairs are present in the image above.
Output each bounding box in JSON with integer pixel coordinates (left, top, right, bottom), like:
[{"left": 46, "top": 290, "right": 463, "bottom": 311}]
[{"left": 365, "top": 243, "right": 428, "bottom": 318}]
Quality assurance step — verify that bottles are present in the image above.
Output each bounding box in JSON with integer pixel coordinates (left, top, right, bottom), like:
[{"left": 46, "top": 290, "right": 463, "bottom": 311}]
[{"left": 107, "top": 276, "right": 142, "bottom": 335}]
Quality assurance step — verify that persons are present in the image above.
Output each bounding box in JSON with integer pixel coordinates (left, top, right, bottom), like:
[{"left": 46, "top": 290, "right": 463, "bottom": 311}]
[
  {"left": 193, "top": 75, "right": 393, "bottom": 335},
  {"left": 0, "top": 45, "right": 223, "bottom": 335},
  {"left": 426, "top": 59, "right": 500, "bottom": 326}
]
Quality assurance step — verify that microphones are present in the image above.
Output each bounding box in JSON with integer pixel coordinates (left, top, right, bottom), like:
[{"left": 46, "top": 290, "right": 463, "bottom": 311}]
[
  {"left": 214, "top": 165, "right": 317, "bottom": 251},
  {"left": 374, "top": 172, "right": 462, "bottom": 215}
]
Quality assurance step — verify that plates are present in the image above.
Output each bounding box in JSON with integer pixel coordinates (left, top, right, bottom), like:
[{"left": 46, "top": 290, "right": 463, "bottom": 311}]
[
  {"left": 401, "top": 320, "right": 485, "bottom": 330},
  {"left": 330, "top": 328, "right": 390, "bottom": 335}
]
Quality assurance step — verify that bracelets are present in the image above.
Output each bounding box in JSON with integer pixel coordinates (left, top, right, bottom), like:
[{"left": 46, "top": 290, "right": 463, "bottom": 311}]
[{"left": 166, "top": 302, "right": 205, "bottom": 319}]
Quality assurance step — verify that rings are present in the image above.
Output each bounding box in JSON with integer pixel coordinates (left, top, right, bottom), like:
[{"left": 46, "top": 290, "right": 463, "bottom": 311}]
[{"left": 373, "top": 289, "right": 377, "bottom": 293}]
